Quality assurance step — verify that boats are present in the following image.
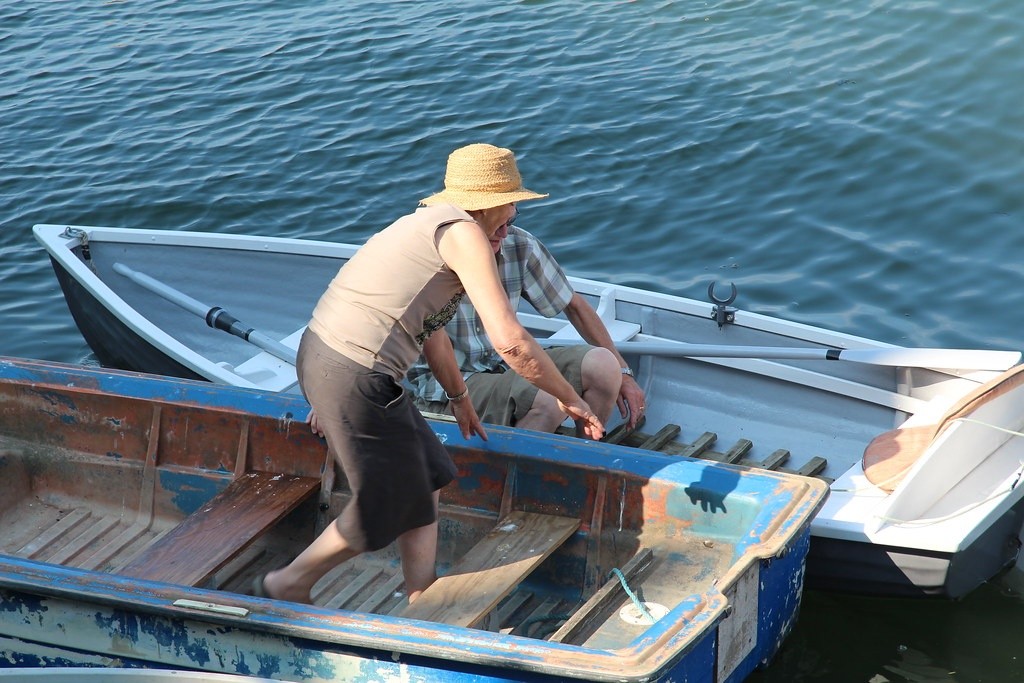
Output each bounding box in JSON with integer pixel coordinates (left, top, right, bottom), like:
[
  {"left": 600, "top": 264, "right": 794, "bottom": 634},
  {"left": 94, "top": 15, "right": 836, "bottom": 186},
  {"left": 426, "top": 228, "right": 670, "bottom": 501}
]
[
  {"left": 28, "top": 220, "right": 1024, "bottom": 602},
  {"left": 1, "top": 357, "right": 832, "bottom": 683}
]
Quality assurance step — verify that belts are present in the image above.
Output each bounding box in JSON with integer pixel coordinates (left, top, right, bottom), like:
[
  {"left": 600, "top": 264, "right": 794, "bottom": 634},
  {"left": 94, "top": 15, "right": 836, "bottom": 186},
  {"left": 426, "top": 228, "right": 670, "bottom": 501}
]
[{"left": 482, "top": 360, "right": 511, "bottom": 374}]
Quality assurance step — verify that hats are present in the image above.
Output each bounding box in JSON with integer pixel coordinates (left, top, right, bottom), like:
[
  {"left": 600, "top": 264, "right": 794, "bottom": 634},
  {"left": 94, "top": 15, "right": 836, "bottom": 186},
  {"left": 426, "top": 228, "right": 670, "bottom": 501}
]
[{"left": 418, "top": 142, "right": 550, "bottom": 211}]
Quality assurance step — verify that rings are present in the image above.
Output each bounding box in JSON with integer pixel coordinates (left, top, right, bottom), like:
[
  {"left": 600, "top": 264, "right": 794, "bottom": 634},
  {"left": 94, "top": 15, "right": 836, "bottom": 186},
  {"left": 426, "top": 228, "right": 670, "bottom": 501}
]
[{"left": 639, "top": 407, "right": 644, "bottom": 410}]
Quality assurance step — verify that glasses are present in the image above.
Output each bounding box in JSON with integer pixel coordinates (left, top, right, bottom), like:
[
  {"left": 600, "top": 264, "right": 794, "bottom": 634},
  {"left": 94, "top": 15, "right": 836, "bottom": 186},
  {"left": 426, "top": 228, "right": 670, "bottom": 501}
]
[{"left": 499, "top": 206, "right": 522, "bottom": 229}]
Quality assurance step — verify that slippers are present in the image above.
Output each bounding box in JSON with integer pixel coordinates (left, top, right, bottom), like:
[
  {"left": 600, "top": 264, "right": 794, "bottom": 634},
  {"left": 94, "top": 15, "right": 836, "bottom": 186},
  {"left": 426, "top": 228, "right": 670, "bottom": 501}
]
[{"left": 251, "top": 574, "right": 269, "bottom": 598}]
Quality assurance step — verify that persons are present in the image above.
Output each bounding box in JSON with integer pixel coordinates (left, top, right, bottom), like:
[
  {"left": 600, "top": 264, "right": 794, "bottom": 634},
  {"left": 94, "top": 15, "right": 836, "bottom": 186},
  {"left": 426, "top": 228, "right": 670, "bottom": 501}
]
[
  {"left": 254, "top": 141, "right": 606, "bottom": 606},
  {"left": 304, "top": 201, "right": 645, "bottom": 442}
]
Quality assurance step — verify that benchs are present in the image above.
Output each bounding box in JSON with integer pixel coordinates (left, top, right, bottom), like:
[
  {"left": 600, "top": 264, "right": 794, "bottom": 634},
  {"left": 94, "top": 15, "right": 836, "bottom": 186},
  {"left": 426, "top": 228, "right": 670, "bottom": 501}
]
[
  {"left": 544, "top": 317, "right": 643, "bottom": 427},
  {"left": 114, "top": 471, "right": 324, "bottom": 591},
  {"left": 234, "top": 325, "right": 303, "bottom": 397},
  {"left": 402, "top": 509, "right": 585, "bottom": 632}
]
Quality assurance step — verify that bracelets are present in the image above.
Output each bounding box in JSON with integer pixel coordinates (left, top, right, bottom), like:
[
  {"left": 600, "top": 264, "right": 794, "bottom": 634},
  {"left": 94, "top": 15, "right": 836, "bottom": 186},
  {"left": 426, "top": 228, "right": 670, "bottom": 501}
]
[
  {"left": 621, "top": 368, "right": 634, "bottom": 377},
  {"left": 444, "top": 384, "right": 469, "bottom": 402}
]
